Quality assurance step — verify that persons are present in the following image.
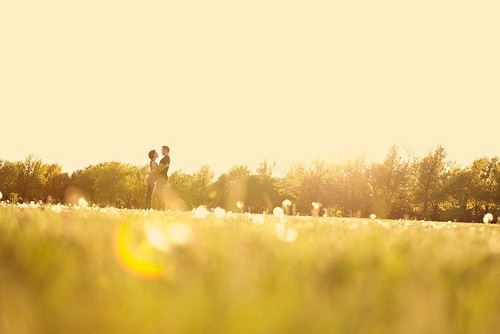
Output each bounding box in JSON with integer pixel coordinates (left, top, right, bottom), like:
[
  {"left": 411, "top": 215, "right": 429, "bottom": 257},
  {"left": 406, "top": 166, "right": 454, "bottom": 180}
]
[
  {"left": 148, "top": 146, "right": 172, "bottom": 210},
  {"left": 145, "top": 149, "right": 170, "bottom": 211}
]
